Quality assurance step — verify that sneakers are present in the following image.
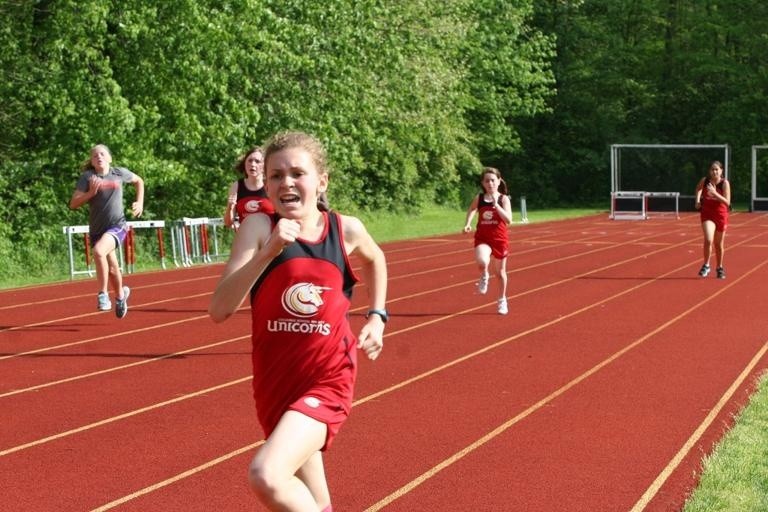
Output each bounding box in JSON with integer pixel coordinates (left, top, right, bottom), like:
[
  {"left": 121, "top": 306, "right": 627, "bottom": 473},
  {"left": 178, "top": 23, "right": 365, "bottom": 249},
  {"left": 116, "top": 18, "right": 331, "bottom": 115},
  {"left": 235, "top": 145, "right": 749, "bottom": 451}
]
[
  {"left": 699, "top": 264, "right": 710, "bottom": 277},
  {"left": 479, "top": 273, "right": 489, "bottom": 294},
  {"left": 717, "top": 267, "right": 725, "bottom": 279},
  {"left": 114, "top": 286, "right": 130, "bottom": 319},
  {"left": 97, "top": 294, "right": 111, "bottom": 311},
  {"left": 497, "top": 297, "right": 508, "bottom": 314}
]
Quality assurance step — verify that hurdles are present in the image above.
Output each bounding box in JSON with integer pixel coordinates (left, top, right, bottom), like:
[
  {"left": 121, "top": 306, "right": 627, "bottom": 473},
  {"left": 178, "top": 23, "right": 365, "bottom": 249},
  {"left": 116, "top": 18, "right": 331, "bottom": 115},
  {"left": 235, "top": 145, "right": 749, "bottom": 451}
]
[
  {"left": 609, "top": 191, "right": 680, "bottom": 220},
  {"left": 62, "top": 218, "right": 241, "bottom": 280}
]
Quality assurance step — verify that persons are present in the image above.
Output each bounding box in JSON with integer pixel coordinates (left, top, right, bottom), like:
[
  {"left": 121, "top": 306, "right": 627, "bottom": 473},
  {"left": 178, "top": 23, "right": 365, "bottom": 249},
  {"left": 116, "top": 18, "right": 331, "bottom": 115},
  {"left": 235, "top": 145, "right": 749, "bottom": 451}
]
[
  {"left": 463, "top": 167, "right": 512, "bottom": 315},
  {"left": 695, "top": 161, "right": 731, "bottom": 279},
  {"left": 209, "top": 132, "right": 388, "bottom": 512},
  {"left": 224, "top": 147, "right": 275, "bottom": 227},
  {"left": 69, "top": 144, "right": 144, "bottom": 319}
]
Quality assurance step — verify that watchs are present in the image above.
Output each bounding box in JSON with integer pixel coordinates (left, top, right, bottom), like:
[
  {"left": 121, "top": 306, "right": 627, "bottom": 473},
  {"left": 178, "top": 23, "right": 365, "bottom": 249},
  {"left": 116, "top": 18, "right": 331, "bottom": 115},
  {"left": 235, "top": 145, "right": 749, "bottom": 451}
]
[{"left": 365, "top": 310, "right": 388, "bottom": 322}]
